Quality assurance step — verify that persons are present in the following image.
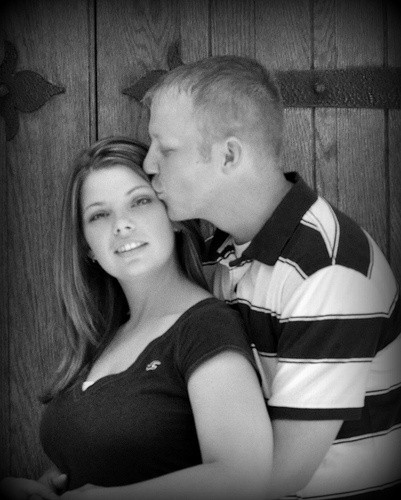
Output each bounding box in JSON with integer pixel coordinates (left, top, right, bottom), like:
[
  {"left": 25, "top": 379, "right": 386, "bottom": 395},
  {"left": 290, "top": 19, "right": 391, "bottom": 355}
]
[
  {"left": 37, "top": 135, "right": 274, "bottom": 500},
  {"left": 140, "top": 55, "right": 400, "bottom": 500}
]
